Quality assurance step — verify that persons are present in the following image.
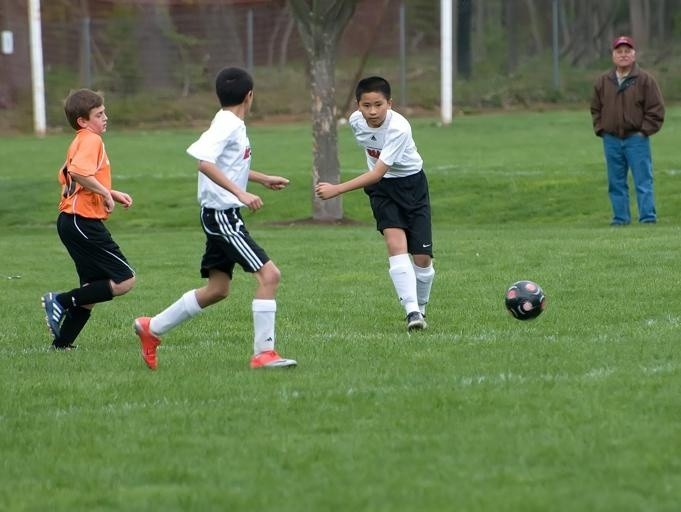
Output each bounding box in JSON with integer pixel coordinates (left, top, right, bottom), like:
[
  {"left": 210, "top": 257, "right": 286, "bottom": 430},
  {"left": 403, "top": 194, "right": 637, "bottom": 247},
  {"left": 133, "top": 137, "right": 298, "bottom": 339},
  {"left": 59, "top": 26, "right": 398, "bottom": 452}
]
[
  {"left": 41, "top": 89, "right": 136, "bottom": 353},
  {"left": 314, "top": 76, "right": 435, "bottom": 331},
  {"left": 590, "top": 37, "right": 666, "bottom": 225},
  {"left": 132, "top": 67, "right": 297, "bottom": 371}
]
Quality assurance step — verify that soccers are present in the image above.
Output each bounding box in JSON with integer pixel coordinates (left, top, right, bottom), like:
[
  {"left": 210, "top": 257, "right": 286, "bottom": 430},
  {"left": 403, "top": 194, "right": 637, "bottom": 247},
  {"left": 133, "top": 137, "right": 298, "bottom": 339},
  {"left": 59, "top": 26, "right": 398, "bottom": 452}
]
[{"left": 503, "top": 279, "right": 546, "bottom": 320}]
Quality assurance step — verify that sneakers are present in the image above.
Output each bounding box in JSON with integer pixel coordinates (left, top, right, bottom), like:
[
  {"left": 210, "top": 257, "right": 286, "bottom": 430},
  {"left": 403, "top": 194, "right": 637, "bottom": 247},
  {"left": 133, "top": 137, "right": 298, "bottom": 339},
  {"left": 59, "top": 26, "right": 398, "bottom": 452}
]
[
  {"left": 48, "top": 345, "right": 75, "bottom": 353},
  {"left": 405, "top": 312, "right": 427, "bottom": 332},
  {"left": 250, "top": 351, "right": 297, "bottom": 368},
  {"left": 41, "top": 293, "right": 66, "bottom": 339},
  {"left": 132, "top": 317, "right": 161, "bottom": 369}
]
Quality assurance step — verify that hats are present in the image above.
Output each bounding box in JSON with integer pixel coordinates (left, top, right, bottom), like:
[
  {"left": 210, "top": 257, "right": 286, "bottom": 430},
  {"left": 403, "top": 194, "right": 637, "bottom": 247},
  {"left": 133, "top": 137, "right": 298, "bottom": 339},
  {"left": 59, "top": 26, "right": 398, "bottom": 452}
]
[{"left": 614, "top": 36, "right": 637, "bottom": 49}]
